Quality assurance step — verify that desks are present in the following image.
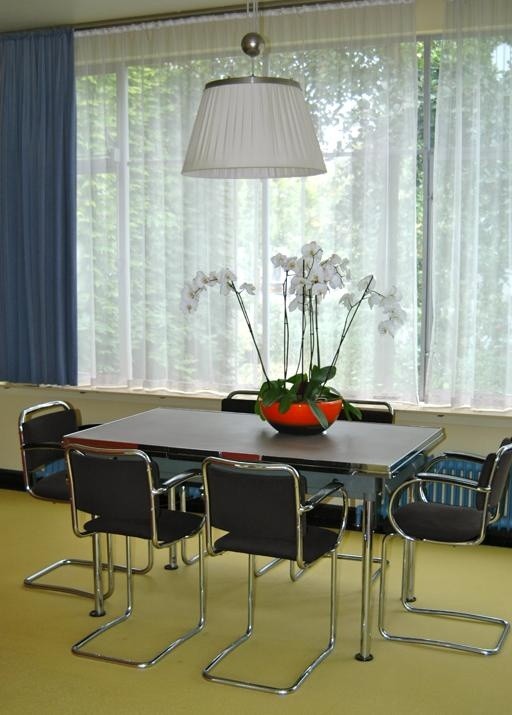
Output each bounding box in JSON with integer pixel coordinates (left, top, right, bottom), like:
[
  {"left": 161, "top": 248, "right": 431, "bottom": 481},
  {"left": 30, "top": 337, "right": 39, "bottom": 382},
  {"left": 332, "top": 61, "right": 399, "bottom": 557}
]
[{"left": 62, "top": 407, "right": 447, "bottom": 662}]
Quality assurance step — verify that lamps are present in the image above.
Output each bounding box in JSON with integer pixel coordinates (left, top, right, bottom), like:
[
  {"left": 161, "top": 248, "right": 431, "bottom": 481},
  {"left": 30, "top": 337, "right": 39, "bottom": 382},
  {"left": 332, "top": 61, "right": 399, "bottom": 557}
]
[{"left": 182, "top": 0, "right": 327, "bottom": 180}]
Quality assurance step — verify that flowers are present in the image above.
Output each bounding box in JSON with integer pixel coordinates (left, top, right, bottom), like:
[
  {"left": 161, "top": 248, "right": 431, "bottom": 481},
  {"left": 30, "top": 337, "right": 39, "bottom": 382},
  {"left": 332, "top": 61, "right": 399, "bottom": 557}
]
[{"left": 180, "top": 241, "right": 406, "bottom": 428}]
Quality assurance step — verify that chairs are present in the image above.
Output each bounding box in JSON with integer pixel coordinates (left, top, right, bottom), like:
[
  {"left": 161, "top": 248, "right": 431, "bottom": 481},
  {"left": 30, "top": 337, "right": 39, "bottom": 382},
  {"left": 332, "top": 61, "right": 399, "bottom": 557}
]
[
  {"left": 377, "top": 437, "right": 511, "bottom": 657},
  {"left": 200, "top": 453, "right": 349, "bottom": 693},
  {"left": 181, "top": 389, "right": 397, "bottom": 586},
  {"left": 63, "top": 442, "right": 208, "bottom": 671},
  {"left": 18, "top": 397, "right": 153, "bottom": 601}
]
